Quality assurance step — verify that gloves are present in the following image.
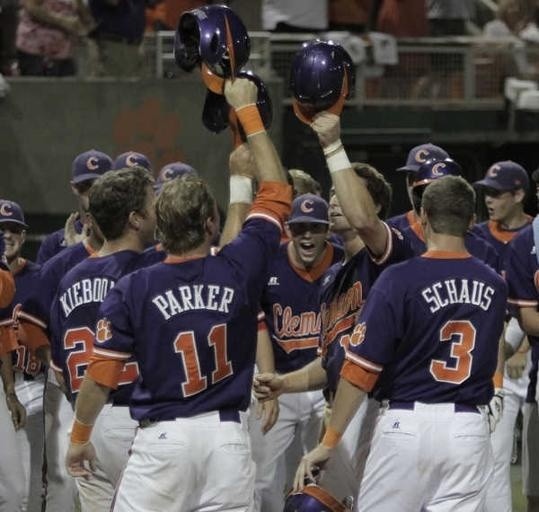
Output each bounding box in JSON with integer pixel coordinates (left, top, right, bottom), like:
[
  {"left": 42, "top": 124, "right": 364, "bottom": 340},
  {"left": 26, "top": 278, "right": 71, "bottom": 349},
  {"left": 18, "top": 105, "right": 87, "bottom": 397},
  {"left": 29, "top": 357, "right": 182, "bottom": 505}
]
[{"left": 483, "top": 371, "right": 505, "bottom": 433}]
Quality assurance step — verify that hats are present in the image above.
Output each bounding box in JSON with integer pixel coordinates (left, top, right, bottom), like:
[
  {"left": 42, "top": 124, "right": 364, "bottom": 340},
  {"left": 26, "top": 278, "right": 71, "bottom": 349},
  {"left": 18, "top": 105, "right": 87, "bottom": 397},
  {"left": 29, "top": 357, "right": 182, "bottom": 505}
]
[
  {"left": 111, "top": 150, "right": 152, "bottom": 174},
  {"left": 71, "top": 148, "right": 114, "bottom": 184},
  {"left": 0, "top": 199, "right": 31, "bottom": 228},
  {"left": 474, "top": 160, "right": 531, "bottom": 192},
  {"left": 286, "top": 192, "right": 330, "bottom": 226},
  {"left": 395, "top": 142, "right": 450, "bottom": 173},
  {"left": 154, "top": 161, "right": 199, "bottom": 189}
]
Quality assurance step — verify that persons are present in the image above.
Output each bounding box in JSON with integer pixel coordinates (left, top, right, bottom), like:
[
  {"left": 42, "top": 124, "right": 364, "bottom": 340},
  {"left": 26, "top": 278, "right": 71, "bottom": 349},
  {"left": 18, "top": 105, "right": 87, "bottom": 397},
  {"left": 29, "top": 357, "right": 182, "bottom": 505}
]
[
  {"left": 65, "top": 77, "right": 293, "bottom": 512},
  {"left": 86, "top": 0, "right": 147, "bottom": 76},
  {"left": 1, "top": 200, "right": 44, "bottom": 511},
  {"left": 502, "top": 168, "right": 539, "bottom": 512},
  {"left": 388, "top": 141, "right": 486, "bottom": 238},
  {"left": 405, "top": 159, "right": 512, "bottom": 432},
  {"left": 147, "top": 1, "right": 172, "bottom": 38},
  {"left": 0, "top": 231, "right": 26, "bottom": 512},
  {"left": 248, "top": 192, "right": 349, "bottom": 512},
  {"left": 37, "top": 149, "right": 113, "bottom": 266},
  {"left": 16, "top": 0, "right": 96, "bottom": 76},
  {"left": 293, "top": 175, "right": 508, "bottom": 512},
  {"left": 473, "top": 160, "right": 537, "bottom": 511},
  {"left": 40, "top": 187, "right": 105, "bottom": 511},
  {"left": 252, "top": 110, "right": 419, "bottom": 512},
  {"left": 260, "top": 0, "right": 539, "bottom": 78},
  {"left": 51, "top": 142, "right": 258, "bottom": 512},
  {"left": 280, "top": 169, "right": 343, "bottom": 244}
]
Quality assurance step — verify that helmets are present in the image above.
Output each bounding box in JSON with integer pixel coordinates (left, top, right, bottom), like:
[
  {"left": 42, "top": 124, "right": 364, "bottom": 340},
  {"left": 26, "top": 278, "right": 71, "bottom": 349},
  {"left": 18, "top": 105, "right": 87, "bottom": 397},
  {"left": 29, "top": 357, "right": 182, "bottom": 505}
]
[
  {"left": 289, "top": 37, "right": 357, "bottom": 125},
  {"left": 201, "top": 69, "right": 273, "bottom": 135},
  {"left": 172, "top": 3, "right": 251, "bottom": 94},
  {"left": 283, "top": 469, "right": 355, "bottom": 512},
  {"left": 413, "top": 155, "right": 465, "bottom": 215}
]
